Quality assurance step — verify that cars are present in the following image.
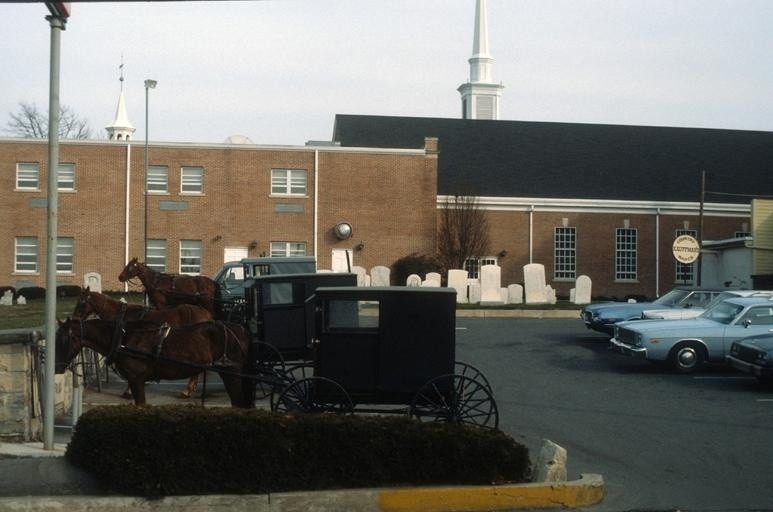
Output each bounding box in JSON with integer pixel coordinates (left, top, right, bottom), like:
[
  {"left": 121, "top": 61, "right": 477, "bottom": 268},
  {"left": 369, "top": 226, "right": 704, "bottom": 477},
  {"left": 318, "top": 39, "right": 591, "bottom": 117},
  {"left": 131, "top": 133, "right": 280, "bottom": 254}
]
[{"left": 580, "top": 287, "right": 773, "bottom": 382}]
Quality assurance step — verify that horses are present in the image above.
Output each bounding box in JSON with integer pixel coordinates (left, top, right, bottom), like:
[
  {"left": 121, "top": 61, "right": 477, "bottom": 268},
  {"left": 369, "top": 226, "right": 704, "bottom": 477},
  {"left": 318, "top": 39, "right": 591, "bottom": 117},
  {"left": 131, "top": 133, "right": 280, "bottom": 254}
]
[
  {"left": 72, "top": 285, "right": 218, "bottom": 400},
  {"left": 117, "top": 256, "right": 221, "bottom": 321},
  {"left": 54, "top": 314, "right": 260, "bottom": 409}
]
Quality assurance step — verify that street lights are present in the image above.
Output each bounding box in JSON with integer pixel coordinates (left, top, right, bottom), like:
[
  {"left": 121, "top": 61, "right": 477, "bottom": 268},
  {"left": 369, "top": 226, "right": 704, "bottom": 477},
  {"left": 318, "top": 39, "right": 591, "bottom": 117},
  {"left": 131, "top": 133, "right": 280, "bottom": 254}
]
[{"left": 142, "top": 79, "right": 157, "bottom": 264}]
